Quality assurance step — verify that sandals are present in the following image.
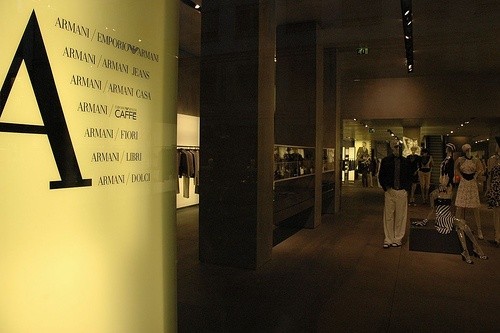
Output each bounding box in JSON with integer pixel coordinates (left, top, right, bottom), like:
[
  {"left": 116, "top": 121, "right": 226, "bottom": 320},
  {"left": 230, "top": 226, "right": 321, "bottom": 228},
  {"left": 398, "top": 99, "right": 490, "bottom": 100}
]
[
  {"left": 392, "top": 242, "right": 401, "bottom": 247},
  {"left": 384, "top": 243, "right": 390, "bottom": 248}
]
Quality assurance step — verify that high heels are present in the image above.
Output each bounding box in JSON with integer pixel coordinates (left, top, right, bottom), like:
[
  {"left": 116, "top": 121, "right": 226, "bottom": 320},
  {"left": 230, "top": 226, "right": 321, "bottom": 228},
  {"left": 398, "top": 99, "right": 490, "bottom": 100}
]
[
  {"left": 473, "top": 249, "right": 488, "bottom": 259},
  {"left": 462, "top": 251, "right": 474, "bottom": 264}
]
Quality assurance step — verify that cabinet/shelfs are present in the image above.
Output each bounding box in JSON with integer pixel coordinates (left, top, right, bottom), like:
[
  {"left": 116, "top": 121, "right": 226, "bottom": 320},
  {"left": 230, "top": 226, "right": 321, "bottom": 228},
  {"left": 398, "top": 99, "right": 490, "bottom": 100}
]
[
  {"left": 322, "top": 147, "right": 335, "bottom": 173},
  {"left": 274, "top": 144, "right": 315, "bottom": 183}
]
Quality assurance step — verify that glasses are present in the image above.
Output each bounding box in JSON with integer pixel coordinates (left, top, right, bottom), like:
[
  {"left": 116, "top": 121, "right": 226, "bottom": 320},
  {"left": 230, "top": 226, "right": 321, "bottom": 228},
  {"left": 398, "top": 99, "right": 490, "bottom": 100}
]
[{"left": 392, "top": 144, "right": 400, "bottom": 149}]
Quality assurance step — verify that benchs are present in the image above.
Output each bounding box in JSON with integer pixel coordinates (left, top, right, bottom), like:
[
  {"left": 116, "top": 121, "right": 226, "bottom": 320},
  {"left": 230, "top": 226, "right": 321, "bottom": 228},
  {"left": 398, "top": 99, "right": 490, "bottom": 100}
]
[{"left": 410, "top": 218, "right": 472, "bottom": 256}]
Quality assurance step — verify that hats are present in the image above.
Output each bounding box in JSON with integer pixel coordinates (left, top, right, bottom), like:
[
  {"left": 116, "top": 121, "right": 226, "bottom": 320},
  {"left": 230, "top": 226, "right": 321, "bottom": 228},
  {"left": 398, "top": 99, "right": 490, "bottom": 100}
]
[
  {"left": 446, "top": 143, "right": 455, "bottom": 151},
  {"left": 461, "top": 145, "right": 471, "bottom": 151}
]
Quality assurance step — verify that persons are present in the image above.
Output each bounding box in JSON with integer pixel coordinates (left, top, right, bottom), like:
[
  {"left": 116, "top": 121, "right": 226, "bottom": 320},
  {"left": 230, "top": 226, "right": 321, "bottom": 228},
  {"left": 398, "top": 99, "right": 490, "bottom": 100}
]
[
  {"left": 357, "top": 142, "right": 368, "bottom": 160},
  {"left": 440, "top": 143, "right": 456, "bottom": 176},
  {"left": 422, "top": 175, "right": 488, "bottom": 264},
  {"left": 485, "top": 142, "right": 500, "bottom": 245},
  {"left": 360, "top": 157, "right": 382, "bottom": 188},
  {"left": 378, "top": 140, "right": 412, "bottom": 248},
  {"left": 274, "top": 147, "right": 313, "bottom": 177},
  {"left": 407, "top": 147, "right": 434, "bottom": 206},
  {"left": 455, "top": 145, "right": 484, "bottom": 239}
]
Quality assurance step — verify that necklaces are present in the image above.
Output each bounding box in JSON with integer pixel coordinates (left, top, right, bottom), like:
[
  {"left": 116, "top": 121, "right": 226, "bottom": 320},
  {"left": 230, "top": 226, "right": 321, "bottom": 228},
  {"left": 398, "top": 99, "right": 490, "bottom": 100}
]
[{"left": 438, "top": 187, "right": 447, "bottom": 193}]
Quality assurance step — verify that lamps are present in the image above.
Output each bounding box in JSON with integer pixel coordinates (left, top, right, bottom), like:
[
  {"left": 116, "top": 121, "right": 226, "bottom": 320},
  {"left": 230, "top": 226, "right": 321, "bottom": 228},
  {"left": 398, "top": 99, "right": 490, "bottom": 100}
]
[{"left": 400, "top": 0, "right": 414, "bottom": 73}]
